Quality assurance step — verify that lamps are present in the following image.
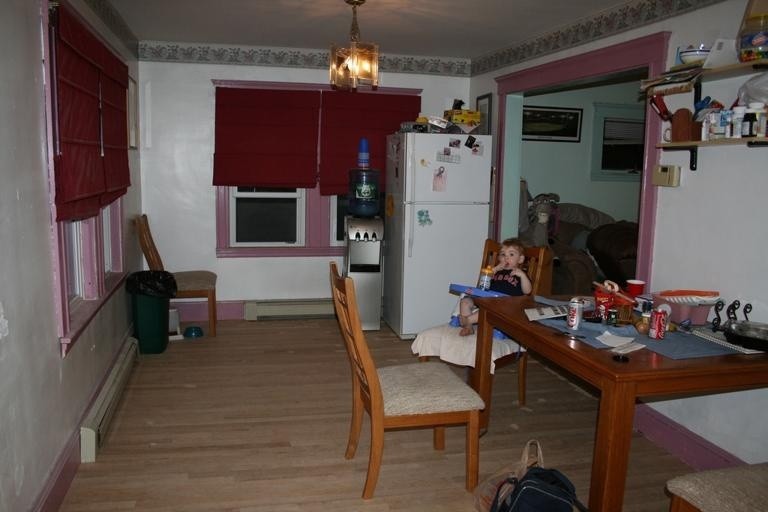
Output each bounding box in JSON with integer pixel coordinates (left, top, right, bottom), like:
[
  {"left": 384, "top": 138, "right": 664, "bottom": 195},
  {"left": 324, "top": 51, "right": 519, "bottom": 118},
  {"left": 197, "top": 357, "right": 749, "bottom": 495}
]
[{"left": 327, "top": 0, "right": 381, "bottom": 93}]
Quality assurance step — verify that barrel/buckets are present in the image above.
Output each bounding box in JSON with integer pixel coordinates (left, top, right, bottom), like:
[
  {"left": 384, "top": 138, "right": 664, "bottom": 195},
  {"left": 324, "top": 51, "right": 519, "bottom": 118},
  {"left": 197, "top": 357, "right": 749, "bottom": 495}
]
[{"left": 347, "top": 167, "right": 383, "bottom": 217}]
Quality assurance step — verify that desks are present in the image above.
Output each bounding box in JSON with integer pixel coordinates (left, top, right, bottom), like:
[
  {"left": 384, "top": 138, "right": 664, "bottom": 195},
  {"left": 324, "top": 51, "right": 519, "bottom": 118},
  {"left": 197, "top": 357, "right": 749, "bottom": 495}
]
[{"left": 469, "top": 292, "right": 766, "bottom": 512}]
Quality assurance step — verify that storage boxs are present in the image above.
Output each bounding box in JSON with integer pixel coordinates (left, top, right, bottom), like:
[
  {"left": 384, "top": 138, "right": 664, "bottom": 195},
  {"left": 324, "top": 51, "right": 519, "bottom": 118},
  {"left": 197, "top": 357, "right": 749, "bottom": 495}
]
[{"left": 652, "top": 293, "right": 712, "bottom": 326}]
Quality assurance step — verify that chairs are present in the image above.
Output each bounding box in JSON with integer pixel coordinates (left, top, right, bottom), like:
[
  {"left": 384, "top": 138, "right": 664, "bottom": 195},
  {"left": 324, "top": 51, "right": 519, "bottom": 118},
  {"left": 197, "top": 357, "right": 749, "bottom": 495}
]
[
  {"left": 130, "top": 214, "right": 218, "bottom": 339},
  {"left": 553, "top": 203, "right": 636, "bottom": 295}
]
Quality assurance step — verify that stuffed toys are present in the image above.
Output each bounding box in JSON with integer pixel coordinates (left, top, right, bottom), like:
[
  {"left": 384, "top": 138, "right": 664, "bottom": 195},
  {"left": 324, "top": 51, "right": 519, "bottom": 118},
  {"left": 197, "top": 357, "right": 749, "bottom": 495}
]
[{"left": 518, "top": 193, "right": 561, "bottom": 267}]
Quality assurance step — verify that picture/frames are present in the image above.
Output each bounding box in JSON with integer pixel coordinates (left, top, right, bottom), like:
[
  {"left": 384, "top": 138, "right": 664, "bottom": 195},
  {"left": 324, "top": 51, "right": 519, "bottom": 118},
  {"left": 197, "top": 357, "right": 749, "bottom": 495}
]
[
  {"left": 522, "top": 105, "right": 584, "bottom": 143},
  {"left": 474, "top": 93, "right": 493, "bottom": 134}
]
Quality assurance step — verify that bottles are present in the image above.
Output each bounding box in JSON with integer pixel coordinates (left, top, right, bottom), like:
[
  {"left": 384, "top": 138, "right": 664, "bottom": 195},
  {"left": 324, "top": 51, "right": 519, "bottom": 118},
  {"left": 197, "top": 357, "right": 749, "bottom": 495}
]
[
  {"left": 480, "top": 265, "right": 491, "bottom": 291},
  {"left": 357, "top": 138, "right": 371, "bottom": 168},
  {"left": 730, "top": 101, "right": 766, "bottom": 138}
]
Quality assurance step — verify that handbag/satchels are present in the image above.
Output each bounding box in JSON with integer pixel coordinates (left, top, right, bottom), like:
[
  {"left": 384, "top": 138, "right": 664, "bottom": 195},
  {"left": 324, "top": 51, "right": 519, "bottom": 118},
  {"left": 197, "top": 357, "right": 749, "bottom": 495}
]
[{"left": 472, "top": 439, "right": 544, "bottom": 512}]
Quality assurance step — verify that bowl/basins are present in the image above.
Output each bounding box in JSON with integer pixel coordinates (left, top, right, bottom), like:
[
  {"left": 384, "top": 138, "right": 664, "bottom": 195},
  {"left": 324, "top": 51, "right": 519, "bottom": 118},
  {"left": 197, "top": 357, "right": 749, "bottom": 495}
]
[{"left": 679, "top": 51, "right": 708, "bottom": 62}]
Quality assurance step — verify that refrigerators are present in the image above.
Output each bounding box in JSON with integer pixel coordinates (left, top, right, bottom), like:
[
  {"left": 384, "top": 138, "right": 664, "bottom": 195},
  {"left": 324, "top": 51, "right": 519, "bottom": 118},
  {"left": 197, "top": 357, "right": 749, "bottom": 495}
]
[{"left": 383, "top": 132, "right": 493, "bottom": 342}]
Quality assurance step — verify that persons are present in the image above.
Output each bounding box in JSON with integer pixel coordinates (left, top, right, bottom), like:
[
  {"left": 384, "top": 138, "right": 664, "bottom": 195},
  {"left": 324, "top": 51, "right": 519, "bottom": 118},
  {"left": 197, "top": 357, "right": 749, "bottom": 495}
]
[{"left": 459, "top": 239, "right": 532, "bottom": 337}]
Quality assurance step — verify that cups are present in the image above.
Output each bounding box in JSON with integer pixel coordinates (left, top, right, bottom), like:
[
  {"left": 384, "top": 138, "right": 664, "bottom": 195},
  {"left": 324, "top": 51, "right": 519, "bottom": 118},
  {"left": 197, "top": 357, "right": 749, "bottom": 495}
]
[{"left": 626, "top": 279, "right": 644, "bottom": 298}]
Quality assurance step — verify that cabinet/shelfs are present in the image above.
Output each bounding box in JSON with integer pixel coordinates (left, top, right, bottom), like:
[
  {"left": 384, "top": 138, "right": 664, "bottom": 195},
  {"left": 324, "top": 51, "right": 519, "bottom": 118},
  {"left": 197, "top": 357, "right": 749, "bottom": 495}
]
[{"left": 655, "top": 58, "right": 768, "bottom": 170}]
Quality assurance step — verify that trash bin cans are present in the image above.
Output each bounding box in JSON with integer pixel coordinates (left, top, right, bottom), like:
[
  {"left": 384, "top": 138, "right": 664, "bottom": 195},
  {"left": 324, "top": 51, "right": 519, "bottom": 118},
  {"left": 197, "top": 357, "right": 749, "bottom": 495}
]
[{"left": 125, "top": 270, "right": 173, "bottom": 355}]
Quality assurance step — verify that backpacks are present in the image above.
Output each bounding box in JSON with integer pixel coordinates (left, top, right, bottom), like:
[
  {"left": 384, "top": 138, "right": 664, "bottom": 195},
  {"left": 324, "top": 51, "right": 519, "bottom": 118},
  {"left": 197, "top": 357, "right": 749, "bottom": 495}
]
[{"left": 490, "top": 467, "right": 589, "bottom": 512}]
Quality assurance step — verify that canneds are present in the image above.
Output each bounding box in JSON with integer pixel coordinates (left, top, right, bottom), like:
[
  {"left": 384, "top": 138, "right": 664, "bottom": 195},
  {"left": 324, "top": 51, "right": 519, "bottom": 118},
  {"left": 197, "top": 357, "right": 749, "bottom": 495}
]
[
  {"left": 566, "top": 301, "right": 582, "bottom": 331},
  {"left": 606, "top": 309, "right": 618, "bottom": 326},
  {"left": 648, "top": 309, "right": 668, "bottom": 339}
]
